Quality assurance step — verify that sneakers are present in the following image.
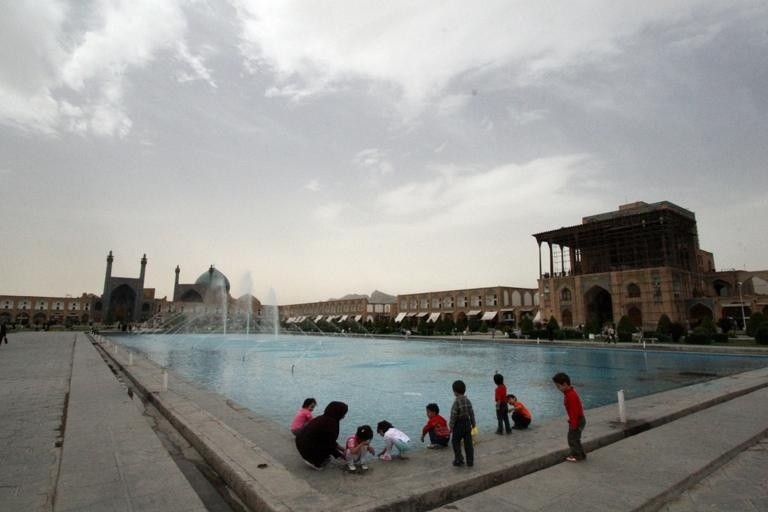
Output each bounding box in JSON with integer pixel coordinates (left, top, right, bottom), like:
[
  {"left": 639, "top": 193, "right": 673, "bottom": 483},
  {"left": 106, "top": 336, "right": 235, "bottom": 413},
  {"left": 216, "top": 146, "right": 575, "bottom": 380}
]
[
  {"left": 360, "top": 464, "right": 368, "bottom": 469},
  {"left": 452, "top": 456, "right": 464, "bottom": 465},
  {"left": 346, "top": 464, "right": 356, "bottom": 471},
  {"left": 564, "top": 453, "right": 586, "bottom": 461},
  {"left": 397, "top": 453, "right": 409, "bottom": 459},
  {"left": 426, "top": 443, "right": 449, "bottom": 449},
  {"left": 380, "top": 452, "right": 392, "bottom": 461},
  {"left": 302, "top": 457, "right": 325, "bottom": 471}
]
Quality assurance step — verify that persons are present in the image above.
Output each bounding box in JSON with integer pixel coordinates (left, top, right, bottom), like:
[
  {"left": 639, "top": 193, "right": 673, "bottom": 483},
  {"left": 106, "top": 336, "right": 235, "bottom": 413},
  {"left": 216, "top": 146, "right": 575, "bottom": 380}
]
[
  {"left": 420, "top": 403, "right": 450, "bottom": 449},
  {"left": 493, "top": 373, "right": 512, "bottom": 435},
  {"left": 376, "top": 421, "right": 411, "bottom": 461},
  {"left": 606, "top": 324, "right": 616, "bottom": 343},
  {"left": 506, "top": 393, "right": 532, "bottom": 430},
  {"left": 345, "top": 425, "right": 375, "bottom": 471},
  {"left": 289, "top": 398, "right": 317, "bottom": 436},
  {"left": 0, "top": 320, "right": 7, "bottom": 345},
  {"left": 294, "top": 401, "right": 346, "bottom": 470},
  {"left": 552, "top": 372, "right": 586, "bottom": 463},
  {"left": 446, "top": 380, "right": 476, "bottom": 467}
]
